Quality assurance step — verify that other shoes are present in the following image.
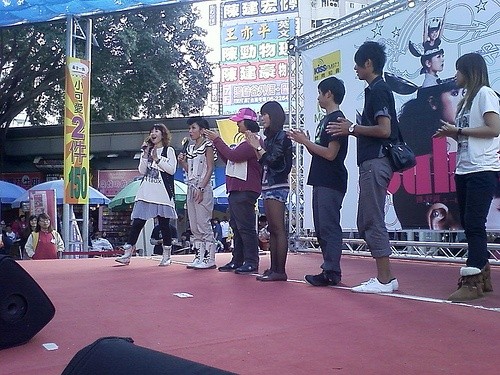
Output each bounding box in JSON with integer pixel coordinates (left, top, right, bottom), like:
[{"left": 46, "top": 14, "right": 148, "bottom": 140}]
[
  {"left": 218, "top": 261, "right": 244, "bottom": 272},
  {"left": 304, "top": 272, "right": 340, "bottom": 287},
  {"left": 256, "top": 268, "right": 288, "bottom": 281},
  {"left": 235, "top": 261, "right": 259, "bottom": 274}
]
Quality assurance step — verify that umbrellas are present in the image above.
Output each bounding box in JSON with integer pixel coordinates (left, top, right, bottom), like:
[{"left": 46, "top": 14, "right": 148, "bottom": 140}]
[{"left": 0, "top": 175, "right": 302, "bottom": 225}]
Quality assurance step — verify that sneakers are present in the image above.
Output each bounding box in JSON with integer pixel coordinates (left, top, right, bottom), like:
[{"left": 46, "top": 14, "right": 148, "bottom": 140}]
[{"left": 351, "top": 277, "right": 399, "bottom": 293}]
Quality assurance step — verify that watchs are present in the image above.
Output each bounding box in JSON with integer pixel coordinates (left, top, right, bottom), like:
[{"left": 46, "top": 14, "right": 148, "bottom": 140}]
[
  {"left": 256, "top": 146, "right": 262, "bottom": 152},
  {"left": 198, "top": 187, "right": 205, "bottom": 193},
  {"left": 348, "top": 123, "right": 357, "bottom": 134}
]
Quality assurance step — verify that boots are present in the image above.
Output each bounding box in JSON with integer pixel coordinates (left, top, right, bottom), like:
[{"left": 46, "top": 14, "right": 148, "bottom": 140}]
[
  {"left": 446, "top": 264, "right": 494, "bottom": 304},
  {"left": 114, "top": 243, "right": 136, "bottom": 265},
  {"left": 186, "top": 242, "right": 217, "bottom": 269},
  {"left": 158, "top": 244, "right": 172, "bottom": 266}
]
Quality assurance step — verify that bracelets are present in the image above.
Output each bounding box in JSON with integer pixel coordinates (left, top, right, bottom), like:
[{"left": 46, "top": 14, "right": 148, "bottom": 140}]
[
  {"left": 156, "top": 159, "right": 160, "bottom": 164},
  {"left": 457, "top": 127, "right": 464, "bottom": 136}
]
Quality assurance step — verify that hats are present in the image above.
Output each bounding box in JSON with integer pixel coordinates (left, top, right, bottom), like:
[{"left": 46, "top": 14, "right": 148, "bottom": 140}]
[{"left": 229, "top": 107, "right": 258, "bottom": 123}]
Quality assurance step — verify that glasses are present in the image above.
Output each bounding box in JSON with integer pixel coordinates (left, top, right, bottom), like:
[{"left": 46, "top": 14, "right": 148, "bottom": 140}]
[{"left": 39, "top": 219, "right": 49, "bottom": 222}]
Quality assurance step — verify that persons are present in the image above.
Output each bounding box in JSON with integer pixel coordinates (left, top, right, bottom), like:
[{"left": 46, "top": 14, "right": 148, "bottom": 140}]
[
  {"left": 204, "top": 108, "right": 264, "bottom": 273},
  {"left": 257, "top": 216, "right": 271, "bottom": 251},
  {"left": 388, "top": 7, "right": 468, "bottom": 231},
  {"left": 210, "top": 216, "right": 235, "bottom": 253},
  {"left": 286, "top": 77, "right": 349, "bottom": 286},
  {"left": 114, "top": 124, "right": 177, "bottom": 266},
  {"left": 0, "top": 214, "right": 39, "bottom": 260},
  {"left": 244, "top": 101, "right": 292, "bottom": 281},
  {"left": 182, "top": 228, "right": 193, "bottom": 243},
  {"left": 324, "top": 43, "right": 402, "bottom": 294},
  {"left": 150, "top": 217, "right": 178, "bottom": 255},
  {"left": 178, "top": 116, "right": 217, "bottom": 270},
  {"left": 432, "top": 54, "right": 500, "bottom": 302},
  {"left": 24, "top": 213, "right": 64, "bottom": 260}
]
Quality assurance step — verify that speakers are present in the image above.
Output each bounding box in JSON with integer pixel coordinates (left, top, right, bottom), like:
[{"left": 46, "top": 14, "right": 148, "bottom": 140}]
[
  {"left": 0, "top": 254, "right": 55, "bottom": 348},
  {"left": 61, "top": 336, "right": 238, "bottom": 375}
]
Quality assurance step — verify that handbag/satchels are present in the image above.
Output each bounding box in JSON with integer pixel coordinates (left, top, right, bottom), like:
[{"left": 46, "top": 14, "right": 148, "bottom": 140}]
[{"left": 385, "top": 142, "right": 414, "bottom": 169}]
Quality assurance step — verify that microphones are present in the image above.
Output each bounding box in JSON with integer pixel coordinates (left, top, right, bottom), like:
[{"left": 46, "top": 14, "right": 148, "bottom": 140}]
[
  {"left": 141, "top": 137, "right": 153, "bottom": 151},
  {"left": 182, "top": 137, "right": 190, "bottom": 154}
]
